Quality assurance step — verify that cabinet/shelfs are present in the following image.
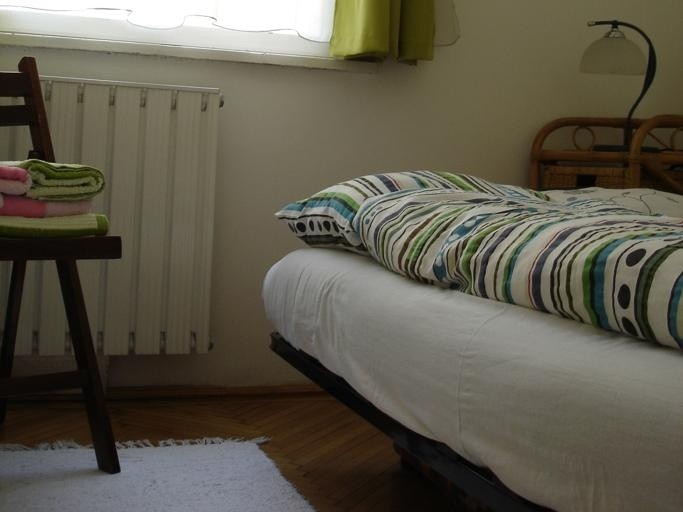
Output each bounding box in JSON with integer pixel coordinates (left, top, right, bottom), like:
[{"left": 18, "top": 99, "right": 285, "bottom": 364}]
[{"left": 526, "top": 112, "right": 683, "bottom": 192}]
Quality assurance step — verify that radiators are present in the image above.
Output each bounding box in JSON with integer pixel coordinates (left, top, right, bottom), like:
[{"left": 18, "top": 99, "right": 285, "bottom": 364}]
[{"left": 0, "top": 70, "right": 223, "bottom": 359}]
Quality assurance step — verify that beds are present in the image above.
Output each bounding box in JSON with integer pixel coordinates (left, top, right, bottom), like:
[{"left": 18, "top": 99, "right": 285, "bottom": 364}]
[{"left": 257, "top": 162, "right": 682, "bottom": 512}]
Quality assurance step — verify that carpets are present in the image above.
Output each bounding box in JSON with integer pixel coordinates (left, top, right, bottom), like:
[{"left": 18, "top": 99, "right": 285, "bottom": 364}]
[{"left": 0, "top": 424, "right": 318, "bottom": 512}]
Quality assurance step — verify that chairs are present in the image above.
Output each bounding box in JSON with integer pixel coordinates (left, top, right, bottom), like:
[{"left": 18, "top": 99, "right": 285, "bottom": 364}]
[{"left": 0, "top": 53, "right": 125, "bottom": 475}]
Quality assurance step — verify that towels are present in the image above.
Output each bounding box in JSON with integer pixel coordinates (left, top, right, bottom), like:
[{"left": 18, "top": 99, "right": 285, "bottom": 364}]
[{"left": 0, "top": 157, "right": 113, "bottom": 238}]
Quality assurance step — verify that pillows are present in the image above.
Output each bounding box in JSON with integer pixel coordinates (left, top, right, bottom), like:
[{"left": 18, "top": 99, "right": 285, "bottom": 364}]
[{"left": 272, "top": 168, "right": 554, "bottom": 261}]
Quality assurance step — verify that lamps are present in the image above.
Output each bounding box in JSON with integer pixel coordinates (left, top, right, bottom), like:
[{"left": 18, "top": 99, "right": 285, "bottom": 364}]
[{"left": 577, "top": 17, "right": 664, "bottom": 154}]
